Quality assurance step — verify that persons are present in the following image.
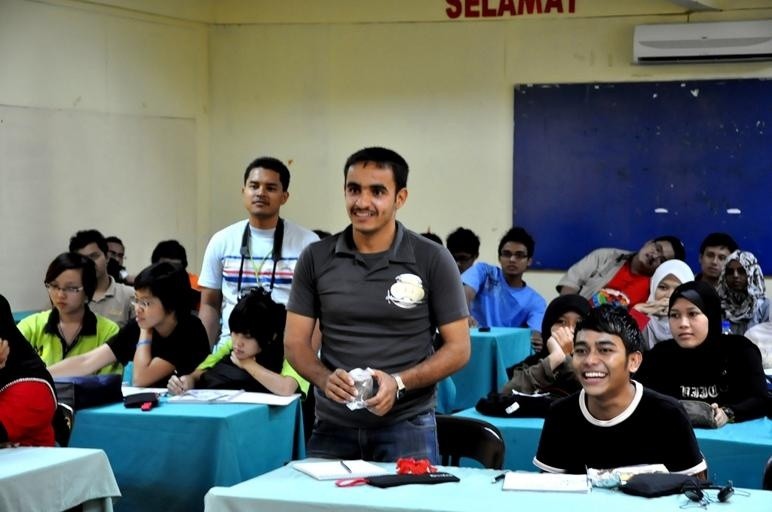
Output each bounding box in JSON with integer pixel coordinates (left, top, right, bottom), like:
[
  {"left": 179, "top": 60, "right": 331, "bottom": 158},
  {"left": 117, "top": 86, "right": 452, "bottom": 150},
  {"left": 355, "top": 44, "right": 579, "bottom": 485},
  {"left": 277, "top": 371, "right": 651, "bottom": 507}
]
[
  {"left": 418, "top": 226, "right": 546, "bottom": 353},
  {"left": 283, "top": 146, "right": 472, "bottom": 466},
  {"left": 501, "top": 230, "right": 771, "bottom": 480},
  {"left": 197, "top": 157, "right": 323, "bottom": 354},
  {"left": 0, "top": 229, "right": 332, "bottom": 450}
]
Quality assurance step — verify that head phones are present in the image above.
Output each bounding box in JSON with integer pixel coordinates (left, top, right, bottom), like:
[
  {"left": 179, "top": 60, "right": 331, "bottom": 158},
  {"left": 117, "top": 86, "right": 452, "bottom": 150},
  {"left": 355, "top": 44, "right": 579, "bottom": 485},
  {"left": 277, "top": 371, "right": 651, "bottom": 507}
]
[{"left": 683, "top": 478, "right": 734, "bottom": 502}]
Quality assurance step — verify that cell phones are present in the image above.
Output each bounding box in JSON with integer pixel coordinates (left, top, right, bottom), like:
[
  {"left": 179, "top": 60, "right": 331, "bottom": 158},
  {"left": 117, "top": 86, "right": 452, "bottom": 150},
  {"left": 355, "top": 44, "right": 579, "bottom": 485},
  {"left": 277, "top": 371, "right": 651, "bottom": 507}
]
[{"left": 479, "top": 326, "right": 490, "bottom": 332}]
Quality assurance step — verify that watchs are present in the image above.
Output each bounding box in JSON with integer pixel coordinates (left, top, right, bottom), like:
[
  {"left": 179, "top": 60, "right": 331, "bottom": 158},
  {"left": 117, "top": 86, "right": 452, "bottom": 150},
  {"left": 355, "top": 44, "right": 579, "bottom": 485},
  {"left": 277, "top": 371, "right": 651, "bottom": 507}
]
[{"left": 389, "top": 372, "right": 407, "bottom": 402}]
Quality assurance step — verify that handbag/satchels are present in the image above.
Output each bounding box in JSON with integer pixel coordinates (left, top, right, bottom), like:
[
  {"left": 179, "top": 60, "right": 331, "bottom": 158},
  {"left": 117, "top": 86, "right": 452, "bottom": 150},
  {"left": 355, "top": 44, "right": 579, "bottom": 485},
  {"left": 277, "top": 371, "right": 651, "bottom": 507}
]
[
  {"left": 199, "top": 350, "right": 272, "bottom": 392},
  {"left": 476, "top": 393, "right": 567, "bottom": 417},
  {"left": 125, "top": 392, "right": 159, "bottom": 410},
  {"left": 54, "top": 373, "right": 124, "bottom": 410},
  {"left": 619, "top": 472, "right": 714, "bottom": 498},
  {"left": 680, "top": 399, "right": 716, "bottom": 429}
]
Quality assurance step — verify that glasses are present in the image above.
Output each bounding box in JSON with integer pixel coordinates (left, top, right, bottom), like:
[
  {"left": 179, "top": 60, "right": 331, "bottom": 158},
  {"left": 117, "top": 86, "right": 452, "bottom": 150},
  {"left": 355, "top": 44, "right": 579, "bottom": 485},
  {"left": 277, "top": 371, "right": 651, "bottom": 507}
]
[
  {"left": 129, "top": 295, "right": 162, "bottom": 311},
  {"left": 655, "top": 240, "right": 666, "bottom": 264},
  {"left": 500, "top": 251, "right": 527, "bottom": 259},
  {"left": 110, "top": 250, "right": 126, "bottom": 258},
  {"left": 45, "top": 281, "right": 84, "bottom": 295}
]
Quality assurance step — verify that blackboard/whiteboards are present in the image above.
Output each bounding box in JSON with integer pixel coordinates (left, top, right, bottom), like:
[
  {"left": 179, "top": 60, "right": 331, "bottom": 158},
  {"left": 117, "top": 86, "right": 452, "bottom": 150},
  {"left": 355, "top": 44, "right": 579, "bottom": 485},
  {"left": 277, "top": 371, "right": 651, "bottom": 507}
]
[{"left": 513, "top": 78, "right": 772, "bottom": 278}]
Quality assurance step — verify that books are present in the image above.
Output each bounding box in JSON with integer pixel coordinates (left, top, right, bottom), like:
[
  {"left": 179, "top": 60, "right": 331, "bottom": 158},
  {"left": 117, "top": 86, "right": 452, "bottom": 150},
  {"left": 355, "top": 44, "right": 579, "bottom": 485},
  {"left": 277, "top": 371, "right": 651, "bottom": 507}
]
[
  {"left": 501, "top": 471, "right": 593, "bottom": 494},
  {"left": 289, "top": 460, "right": 388, "bottom": 481}
]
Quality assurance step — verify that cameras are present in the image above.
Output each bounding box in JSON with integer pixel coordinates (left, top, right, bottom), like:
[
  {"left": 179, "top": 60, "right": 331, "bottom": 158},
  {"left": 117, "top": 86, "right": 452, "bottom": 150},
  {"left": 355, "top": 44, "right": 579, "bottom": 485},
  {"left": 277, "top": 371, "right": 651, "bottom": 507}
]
[{"left": 237, "top": 286, "right": 274, "bottom": 302}]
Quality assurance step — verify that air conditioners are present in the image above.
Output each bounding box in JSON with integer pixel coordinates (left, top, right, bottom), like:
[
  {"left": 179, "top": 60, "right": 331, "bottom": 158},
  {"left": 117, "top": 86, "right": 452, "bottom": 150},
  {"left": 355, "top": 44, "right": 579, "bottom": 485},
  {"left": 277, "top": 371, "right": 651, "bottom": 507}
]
[{"left": 632, "top": 19, "right": 772, "bottom": 65}]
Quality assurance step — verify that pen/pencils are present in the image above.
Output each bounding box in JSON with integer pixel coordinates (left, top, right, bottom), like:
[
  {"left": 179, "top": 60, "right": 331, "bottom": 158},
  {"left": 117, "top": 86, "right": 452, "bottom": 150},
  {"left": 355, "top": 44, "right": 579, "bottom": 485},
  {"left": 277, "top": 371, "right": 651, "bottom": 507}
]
[
  {"left": 492, "top": 471, "right": 509, "bottom": 483},
  {"left": 173, "top": 370, "right": 184, "bottom": 392},
  {"left": 341, "top": 461, "right": 352, "bottom": 473}
]
